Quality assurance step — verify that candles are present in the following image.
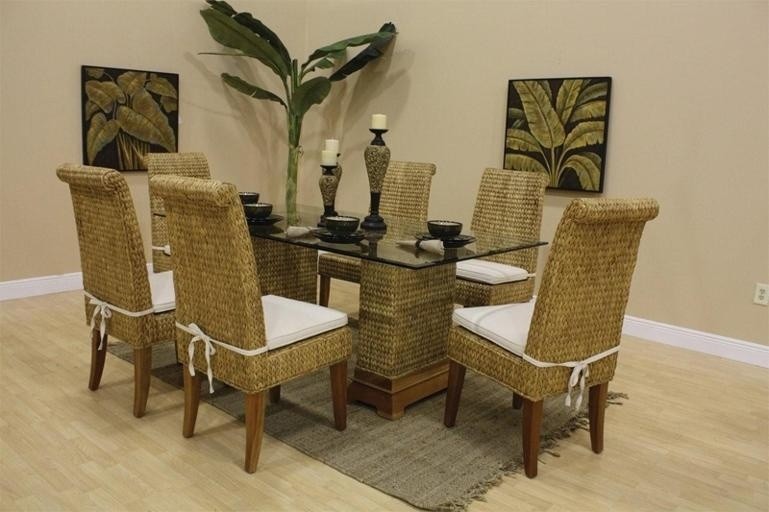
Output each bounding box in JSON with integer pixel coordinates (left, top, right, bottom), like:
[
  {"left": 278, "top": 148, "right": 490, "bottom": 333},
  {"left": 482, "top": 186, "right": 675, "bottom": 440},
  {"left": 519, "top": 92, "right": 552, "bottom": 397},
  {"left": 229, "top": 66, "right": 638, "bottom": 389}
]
[
  {"left": 371, "top": 113, "right": 388, "bottom": 129},
  {"left": 324, "top": 138, "right": 339, "bottom": 155},
  {"left": 320, "top": 151, "right": 338, "bottom": 166},
  {"left": 148, "top": 152, "right": 210, "bottom": 273}
]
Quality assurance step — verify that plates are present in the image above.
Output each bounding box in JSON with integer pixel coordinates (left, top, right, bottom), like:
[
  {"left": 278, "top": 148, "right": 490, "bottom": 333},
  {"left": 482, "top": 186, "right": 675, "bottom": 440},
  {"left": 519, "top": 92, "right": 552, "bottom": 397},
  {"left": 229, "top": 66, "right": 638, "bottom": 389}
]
[
  {"left": 412, "top": 235, "right": 476, "bottom": 248},
  {"left": 312, "top": 229, "right": 366, "bottom": 242},
  {"left": 247, "top": 215, "right": 283, "bottom": 225}
]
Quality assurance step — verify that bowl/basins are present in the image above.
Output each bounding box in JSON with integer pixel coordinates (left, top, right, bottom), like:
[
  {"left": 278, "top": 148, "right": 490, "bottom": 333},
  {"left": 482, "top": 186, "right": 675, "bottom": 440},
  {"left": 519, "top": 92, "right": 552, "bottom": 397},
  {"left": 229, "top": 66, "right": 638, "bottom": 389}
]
[
  {"left": 243, "top": 203, "right": 273, "bottom": 218},
  {"left": 323, "top": 215, "right": 360, "bottom": 234},
  {"left": 426, "top": 221, "right": 463, "bottom": 239},
  {"left": 238, "top": 192, "right": 260, "bottom": 204}
]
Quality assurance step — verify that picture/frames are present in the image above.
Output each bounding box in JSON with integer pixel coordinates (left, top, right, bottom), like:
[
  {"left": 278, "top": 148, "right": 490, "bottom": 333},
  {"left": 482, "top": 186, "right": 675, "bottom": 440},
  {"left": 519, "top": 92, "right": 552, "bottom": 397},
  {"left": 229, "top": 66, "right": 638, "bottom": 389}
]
[
  {"left": 501, "top": 77, "right": 611, "bottom": 192},
  {"left": 81, "top": 64, "right": 179, "bottom": 171}
]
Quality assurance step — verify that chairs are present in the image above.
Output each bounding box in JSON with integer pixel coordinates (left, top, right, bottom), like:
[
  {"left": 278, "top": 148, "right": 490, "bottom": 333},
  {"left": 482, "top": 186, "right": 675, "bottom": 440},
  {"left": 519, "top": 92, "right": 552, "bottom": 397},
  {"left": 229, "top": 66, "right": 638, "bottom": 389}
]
[
  {"left": 377, "top": 160, "right": 436, "bottom": 235},
  {"left": 150, "top": 174, "right": 351, "bottom": 475},
  {"left": 55, "top": 163, "right": 177, "bottom": 418},
  {"left": 456, "top": 167, "right": 549, "bottom": 304},
  {"left": 444, "top": 198, "right": 660, "bottom": 479}
]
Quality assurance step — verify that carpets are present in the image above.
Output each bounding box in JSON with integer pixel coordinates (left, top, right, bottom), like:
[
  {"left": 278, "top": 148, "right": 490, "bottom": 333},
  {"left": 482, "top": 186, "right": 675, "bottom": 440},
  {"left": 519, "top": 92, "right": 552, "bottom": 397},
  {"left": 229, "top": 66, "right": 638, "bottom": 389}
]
[{"left": 104, "top": 337, "right": 631, "bottom": 510}]
